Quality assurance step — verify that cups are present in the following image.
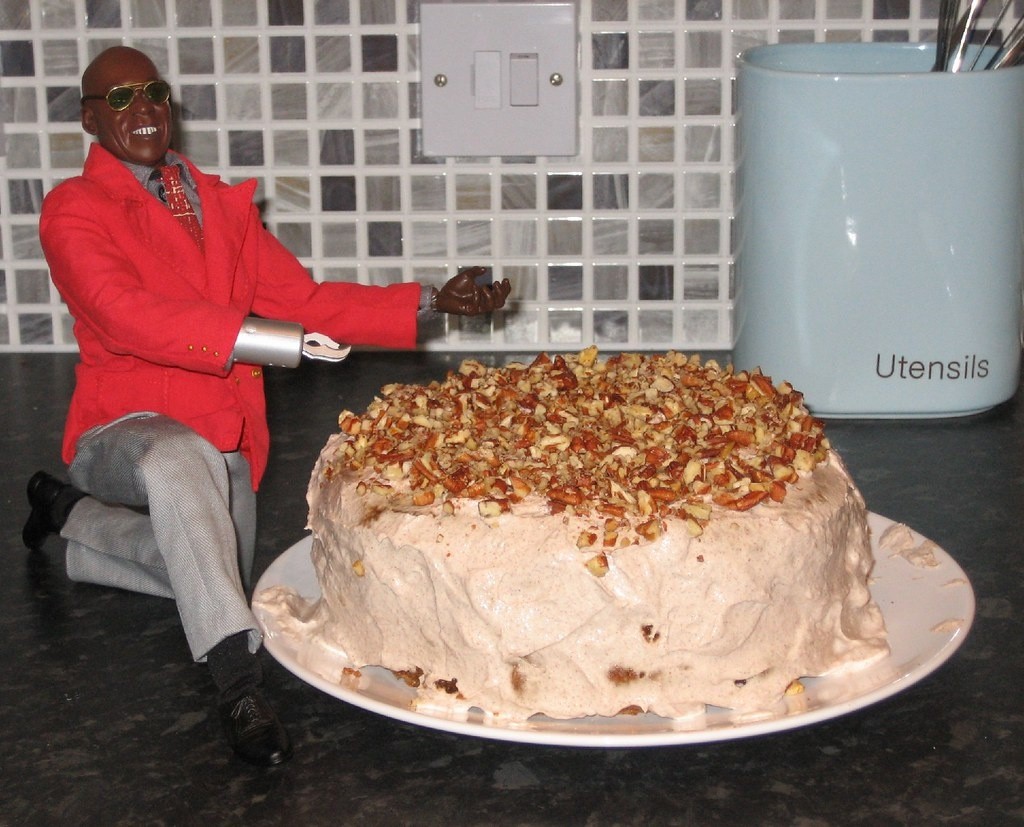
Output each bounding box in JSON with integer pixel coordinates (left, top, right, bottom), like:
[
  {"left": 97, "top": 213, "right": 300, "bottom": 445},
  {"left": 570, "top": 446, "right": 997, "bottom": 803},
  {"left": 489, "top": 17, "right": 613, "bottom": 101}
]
[{"left": 725, "top": 43, "right": 1022, "bottom": 420}]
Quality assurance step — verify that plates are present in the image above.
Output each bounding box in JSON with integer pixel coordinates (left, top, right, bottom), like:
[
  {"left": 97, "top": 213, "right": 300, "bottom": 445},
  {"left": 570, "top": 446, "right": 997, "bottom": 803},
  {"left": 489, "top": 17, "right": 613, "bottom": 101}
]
[{"left": 251, "top": 511, "right": 974, "bottom": 749}]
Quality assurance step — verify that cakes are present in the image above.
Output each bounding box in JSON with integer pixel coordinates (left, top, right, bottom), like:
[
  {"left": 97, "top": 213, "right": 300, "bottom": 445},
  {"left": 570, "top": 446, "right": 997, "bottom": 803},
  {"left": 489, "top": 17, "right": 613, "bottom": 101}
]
[{"left": 252, "top": 345, "right": 889, "bottom": 725}]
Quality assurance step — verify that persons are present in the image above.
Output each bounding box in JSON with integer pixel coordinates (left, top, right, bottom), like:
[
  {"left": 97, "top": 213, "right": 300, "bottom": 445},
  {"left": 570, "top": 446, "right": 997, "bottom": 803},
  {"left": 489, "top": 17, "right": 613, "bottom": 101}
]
[{"left": 19, "top": 45, "right": 512, "bottom": 768}]
